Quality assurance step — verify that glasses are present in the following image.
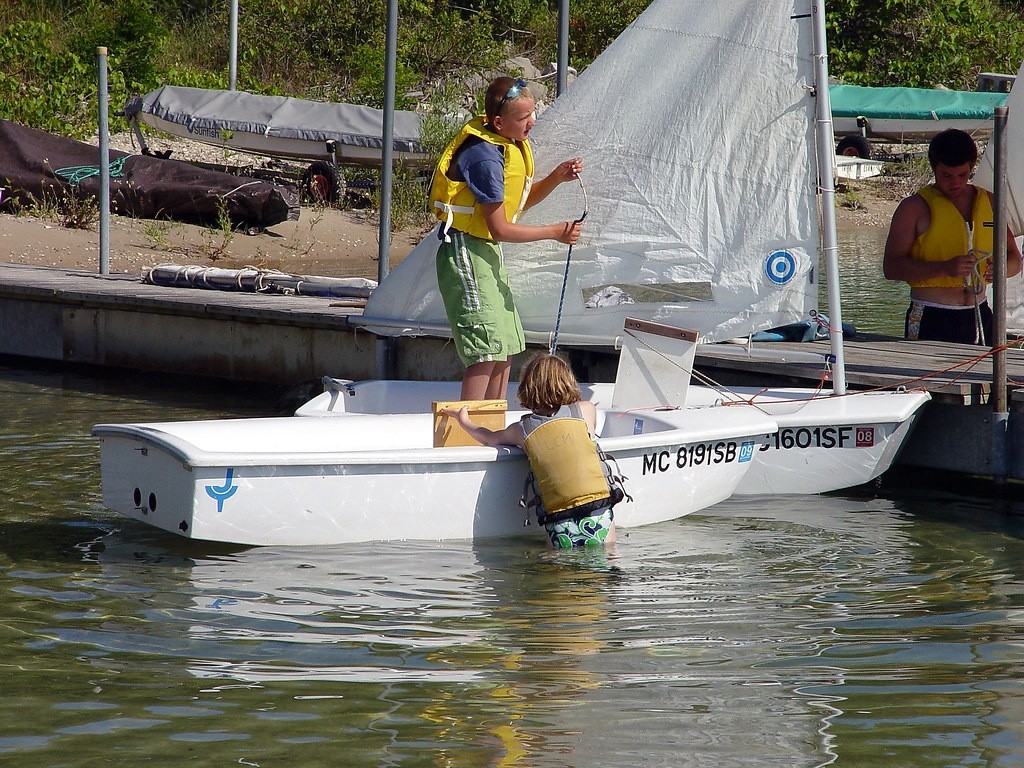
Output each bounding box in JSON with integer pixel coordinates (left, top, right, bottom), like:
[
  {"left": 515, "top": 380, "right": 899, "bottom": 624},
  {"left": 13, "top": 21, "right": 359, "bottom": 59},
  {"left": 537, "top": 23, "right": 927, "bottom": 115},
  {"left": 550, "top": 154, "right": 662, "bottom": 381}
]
[{"left": 492, "top": 79, "right": 528, "bottom": 119}]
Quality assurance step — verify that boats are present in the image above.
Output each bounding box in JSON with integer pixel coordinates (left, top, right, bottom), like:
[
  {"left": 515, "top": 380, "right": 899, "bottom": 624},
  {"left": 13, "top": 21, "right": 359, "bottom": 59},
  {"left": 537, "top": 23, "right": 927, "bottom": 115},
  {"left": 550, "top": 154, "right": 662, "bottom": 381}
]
[
  {"left": 113, "top": 85, "right": 471, "bottom": 172},
  {"left": 89, "top": 315, "right": 778, "bottom": 549},
  {"left": 827, "top": 83, "right": 1011, "bottom": 142}
]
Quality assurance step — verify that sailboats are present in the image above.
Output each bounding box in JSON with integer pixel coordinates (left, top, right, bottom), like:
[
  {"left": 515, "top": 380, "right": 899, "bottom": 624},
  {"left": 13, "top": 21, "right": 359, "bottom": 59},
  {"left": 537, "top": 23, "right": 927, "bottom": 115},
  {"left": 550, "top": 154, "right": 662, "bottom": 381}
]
[{"left": 290, "top": 0, "right": 935, "bottom": 499}]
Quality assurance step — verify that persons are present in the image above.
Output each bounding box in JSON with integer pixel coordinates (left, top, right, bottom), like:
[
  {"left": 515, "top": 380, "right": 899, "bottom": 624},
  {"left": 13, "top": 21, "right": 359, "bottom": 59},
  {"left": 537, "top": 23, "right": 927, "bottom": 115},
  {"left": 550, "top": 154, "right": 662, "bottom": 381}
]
[
  {"left": 883, "top": 128, "right": 1024, "bottom": 347},
  {"left": 443, "top": 354, "right": 616, "bottom": 548},
  {"left": 426, "top": 77, "right": 582, "bottom": 400}
]
